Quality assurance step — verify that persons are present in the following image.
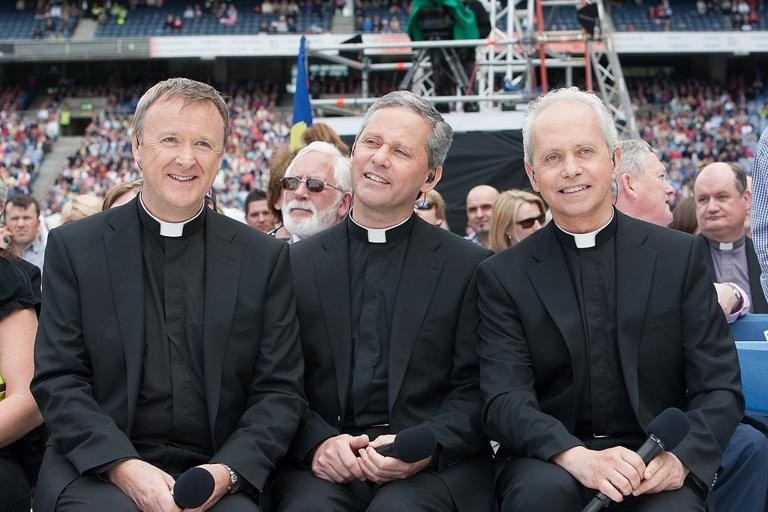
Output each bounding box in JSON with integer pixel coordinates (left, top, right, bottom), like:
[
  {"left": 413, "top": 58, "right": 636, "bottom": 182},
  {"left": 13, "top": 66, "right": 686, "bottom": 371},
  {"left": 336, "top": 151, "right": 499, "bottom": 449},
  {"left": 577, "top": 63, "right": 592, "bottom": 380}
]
[
  {"left": 690, "top": 417, "right": 766, "bottom": 512},
  {"left": 1, "top": 1, "right": 767, "bottom": 324},
  {"left": 31, "top": 73, "right": 308, "bottom": 511},
  {"left": 255, "top": 89, "right": 493, "bottom": 512},
  {"left": 1, "top": 249, "right": 50, "bottom": 512},
  {"left": 471, "top": 83, "right": 747, "bottom": 512}
]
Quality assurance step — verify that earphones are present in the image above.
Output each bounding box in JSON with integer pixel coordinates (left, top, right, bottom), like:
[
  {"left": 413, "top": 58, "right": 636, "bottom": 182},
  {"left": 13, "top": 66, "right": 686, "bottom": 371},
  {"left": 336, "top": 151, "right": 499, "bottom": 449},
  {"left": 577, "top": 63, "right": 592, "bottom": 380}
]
[
  {"left": 137, "top": 139, "right": 139, "bottom": 145},
  {"left": 427, "top": 176, "right": 435, "bottom": 182},
  {"left": 612, "top": 153, "right": 615, "bottom": 166},
  {"left": 507, "top": 235, "right": 511, "bottom": 245}
]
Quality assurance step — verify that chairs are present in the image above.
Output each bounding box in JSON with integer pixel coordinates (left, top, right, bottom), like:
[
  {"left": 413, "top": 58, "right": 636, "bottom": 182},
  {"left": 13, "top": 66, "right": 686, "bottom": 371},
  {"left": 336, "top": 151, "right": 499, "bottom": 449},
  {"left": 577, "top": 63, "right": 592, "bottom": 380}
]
[
  {"left": 728, "top": 312, "right": 767, "bottom": 343},
  {"left": 727, "top": 340, "right": 766, "bottom": 422}
]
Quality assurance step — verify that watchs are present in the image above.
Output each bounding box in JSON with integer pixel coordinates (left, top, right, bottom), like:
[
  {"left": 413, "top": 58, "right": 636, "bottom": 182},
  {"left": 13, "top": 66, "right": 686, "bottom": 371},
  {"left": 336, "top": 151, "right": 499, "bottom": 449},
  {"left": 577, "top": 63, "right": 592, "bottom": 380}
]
[{"left": 221, "top": 463, "right": 241, "bottom": 496}]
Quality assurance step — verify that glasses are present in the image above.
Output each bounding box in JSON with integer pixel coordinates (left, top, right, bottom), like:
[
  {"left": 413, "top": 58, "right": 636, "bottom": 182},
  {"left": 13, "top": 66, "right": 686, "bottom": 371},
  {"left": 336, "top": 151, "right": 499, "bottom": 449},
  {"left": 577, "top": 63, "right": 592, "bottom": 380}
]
[
  {"left": 516, "top": 214, "right": 545, "bottom": 229},
  {"left": 415, "top": 200, "right": 436, "bottom": 210},
  {"left": 280, "top": 176, "right": 342, "bottom": 193}
]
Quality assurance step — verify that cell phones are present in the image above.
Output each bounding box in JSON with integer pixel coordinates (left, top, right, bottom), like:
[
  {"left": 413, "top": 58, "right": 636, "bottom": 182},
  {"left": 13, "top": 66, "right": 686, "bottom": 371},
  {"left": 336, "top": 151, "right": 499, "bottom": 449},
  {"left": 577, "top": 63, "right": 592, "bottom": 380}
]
[{"left": 0, "top": 215, "right": 12, "bottom": 245}]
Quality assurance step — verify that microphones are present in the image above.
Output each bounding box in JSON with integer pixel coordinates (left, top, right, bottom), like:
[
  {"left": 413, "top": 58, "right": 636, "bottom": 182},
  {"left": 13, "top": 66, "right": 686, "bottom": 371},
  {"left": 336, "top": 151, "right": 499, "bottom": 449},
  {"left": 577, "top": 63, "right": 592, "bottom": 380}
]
[
  {"left": 581, "top": 407, "right": 691, "bottom": 512},
  {"left": 169, "top": 467, "right": 215, "bottom": 509},
  {"left": 374, "top": 425, "right": 436, "bottom": 463}
]
